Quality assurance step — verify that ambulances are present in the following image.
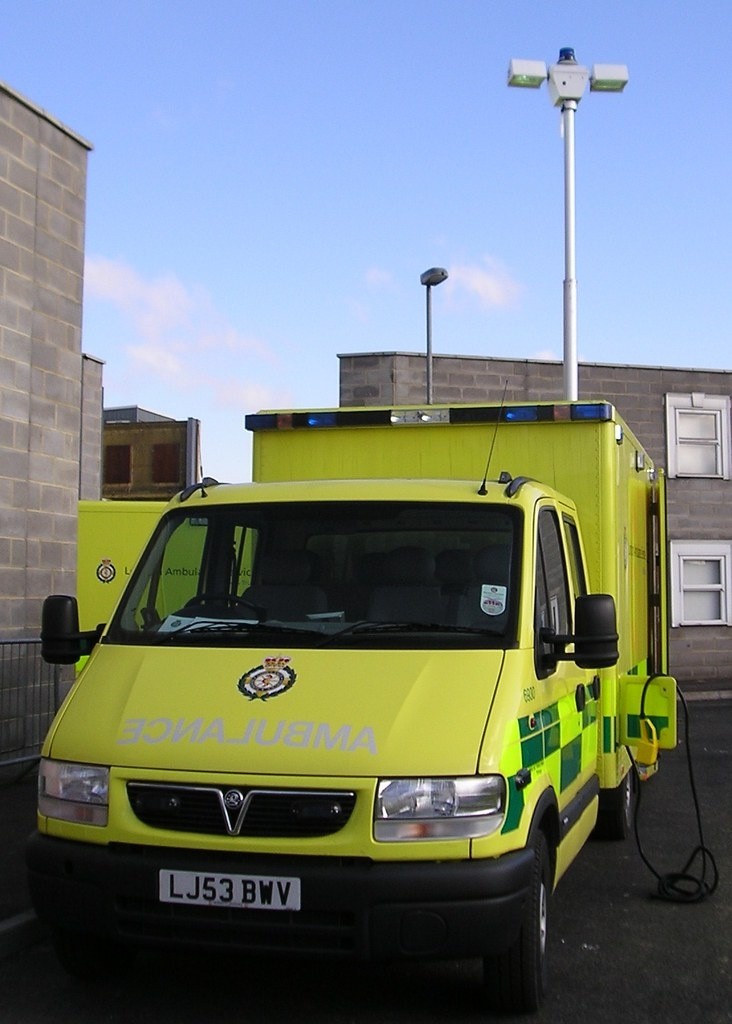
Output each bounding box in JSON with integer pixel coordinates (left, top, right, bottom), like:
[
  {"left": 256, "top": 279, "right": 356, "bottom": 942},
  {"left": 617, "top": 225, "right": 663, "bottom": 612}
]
[{"left": 19, "top": 400, "right": 681, "bottom": 1017}]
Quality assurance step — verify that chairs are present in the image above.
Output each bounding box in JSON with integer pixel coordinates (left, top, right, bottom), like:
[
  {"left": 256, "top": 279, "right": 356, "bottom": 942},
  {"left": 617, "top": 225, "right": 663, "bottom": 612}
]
[{"left": 240, "top": 544, "right": 511, "bottom": 632}]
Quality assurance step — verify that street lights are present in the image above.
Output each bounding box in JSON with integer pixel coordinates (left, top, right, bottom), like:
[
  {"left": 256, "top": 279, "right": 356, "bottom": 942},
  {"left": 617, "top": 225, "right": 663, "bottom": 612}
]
[
  {"left": 509, "top": 46, "right": 629, "bottom": 392},
  {"left": 415, "top": 267, "right": 446, "bottom": 407}
]
